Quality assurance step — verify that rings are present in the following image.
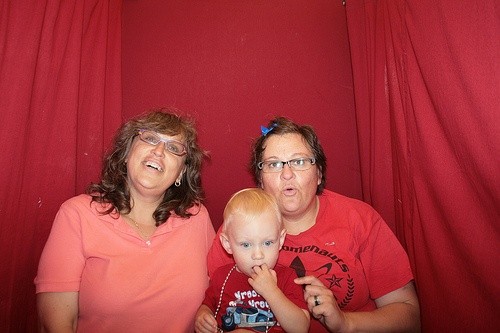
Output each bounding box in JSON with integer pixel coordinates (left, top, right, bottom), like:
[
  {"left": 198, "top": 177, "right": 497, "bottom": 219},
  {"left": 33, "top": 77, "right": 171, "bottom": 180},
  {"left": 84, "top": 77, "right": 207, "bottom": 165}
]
[{"left": 314, "top": 296, "right": 321, "bottom": 306}]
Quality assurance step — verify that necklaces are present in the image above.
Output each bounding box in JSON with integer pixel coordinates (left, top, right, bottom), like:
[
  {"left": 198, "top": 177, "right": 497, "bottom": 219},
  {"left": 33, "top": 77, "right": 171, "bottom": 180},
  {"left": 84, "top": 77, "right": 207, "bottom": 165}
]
[
  {"left": 127, "top": 213, "right": 162, "bottom": 245},
  {"left": 215, "top": 264, "right": 272, "bottom": 333}
]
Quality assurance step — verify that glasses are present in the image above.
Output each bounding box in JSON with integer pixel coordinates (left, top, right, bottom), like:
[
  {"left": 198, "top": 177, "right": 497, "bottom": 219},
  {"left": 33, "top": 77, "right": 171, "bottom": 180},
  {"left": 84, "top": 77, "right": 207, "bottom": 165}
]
[
  {"left": 137, "top": 128, "right": 190, "bottom": 157},
  {"left": 257, "top": 157, "right": 317, "bottom": 173}
]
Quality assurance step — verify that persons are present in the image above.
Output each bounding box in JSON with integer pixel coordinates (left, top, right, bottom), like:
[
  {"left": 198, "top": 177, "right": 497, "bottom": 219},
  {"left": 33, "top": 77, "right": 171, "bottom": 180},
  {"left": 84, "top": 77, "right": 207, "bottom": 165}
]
[
  {"left": 205, "top": 115, "right": 422, "bottom": 333},
  {"left": 33, "top": 106, "right": 217, "bottom": 333},
  {"left": 193, "top": 188, "right": 315, "bottom": 333}
]
